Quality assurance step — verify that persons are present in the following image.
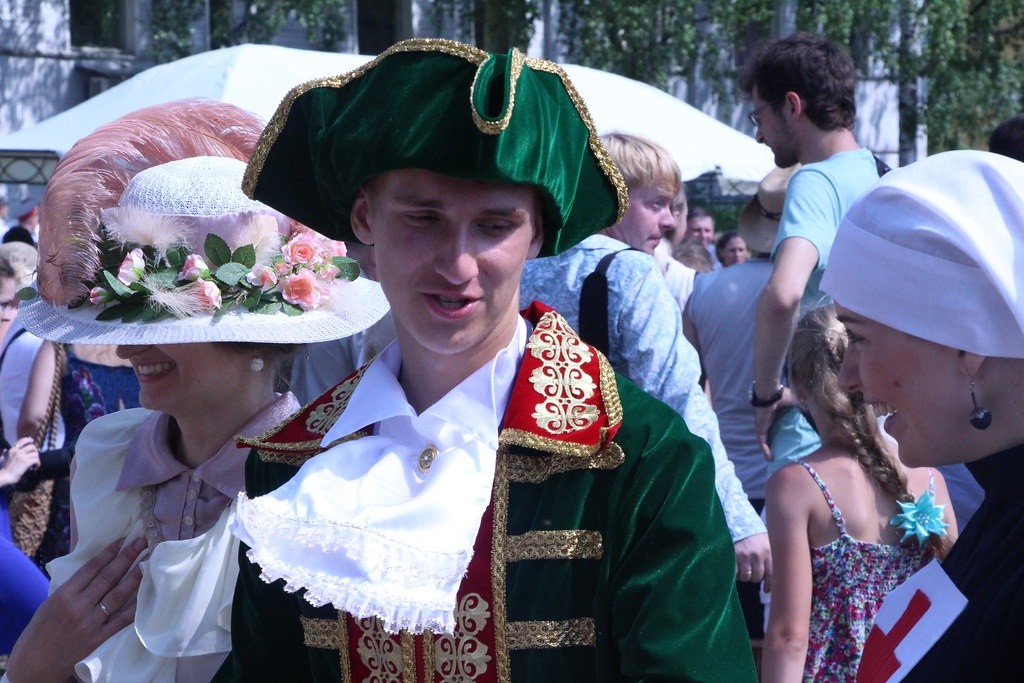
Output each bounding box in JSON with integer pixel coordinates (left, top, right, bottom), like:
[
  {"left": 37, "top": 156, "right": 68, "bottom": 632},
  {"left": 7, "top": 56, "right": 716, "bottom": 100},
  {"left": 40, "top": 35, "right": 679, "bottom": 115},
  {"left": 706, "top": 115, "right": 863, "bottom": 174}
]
[
  {"left": 758, "top": 309, "right": 960, "bottom": 683},
  {"left": 4, "top": 98, "right": 388, "bottom": 683},
  {"left": 826, "top": 147, "right": 1024, "bottom": 683},
  {"left": 512, "top": 31, "right": 885, "bottom": 650},
  {"left": 2, "top": 197, "right": 142, "bottom": 577},
  {"left": 209, "top": 41, "right": 760, "bottom": 683}
]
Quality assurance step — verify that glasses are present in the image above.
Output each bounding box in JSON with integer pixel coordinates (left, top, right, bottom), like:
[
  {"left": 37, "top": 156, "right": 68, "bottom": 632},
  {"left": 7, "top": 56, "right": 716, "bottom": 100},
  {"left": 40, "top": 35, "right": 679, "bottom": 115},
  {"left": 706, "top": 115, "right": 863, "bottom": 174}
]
[{"left": 748, "top": 99, "right": 778, "bottom": 126}]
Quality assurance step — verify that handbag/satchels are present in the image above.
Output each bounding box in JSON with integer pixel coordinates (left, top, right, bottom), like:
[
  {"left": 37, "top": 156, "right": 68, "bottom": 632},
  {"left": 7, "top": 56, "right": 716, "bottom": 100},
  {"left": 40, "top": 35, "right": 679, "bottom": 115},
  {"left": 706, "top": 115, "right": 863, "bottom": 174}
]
[{"left": 3, "top": 445, "right": 76, "bottom": 573}]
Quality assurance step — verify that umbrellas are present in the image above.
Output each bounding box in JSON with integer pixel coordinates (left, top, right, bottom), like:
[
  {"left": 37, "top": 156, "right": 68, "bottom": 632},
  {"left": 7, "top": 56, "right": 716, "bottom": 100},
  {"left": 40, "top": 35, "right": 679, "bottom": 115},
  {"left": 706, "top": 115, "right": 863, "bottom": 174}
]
[{"left": 2, "top": 42, "right": 383, "bottom": 154}]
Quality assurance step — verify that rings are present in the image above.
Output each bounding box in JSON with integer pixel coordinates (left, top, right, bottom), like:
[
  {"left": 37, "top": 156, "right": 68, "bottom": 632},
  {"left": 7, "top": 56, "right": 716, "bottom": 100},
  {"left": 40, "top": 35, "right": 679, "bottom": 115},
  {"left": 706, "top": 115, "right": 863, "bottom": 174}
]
[{"left": 98, "top": 601, "right": 111, "bottom": 616}]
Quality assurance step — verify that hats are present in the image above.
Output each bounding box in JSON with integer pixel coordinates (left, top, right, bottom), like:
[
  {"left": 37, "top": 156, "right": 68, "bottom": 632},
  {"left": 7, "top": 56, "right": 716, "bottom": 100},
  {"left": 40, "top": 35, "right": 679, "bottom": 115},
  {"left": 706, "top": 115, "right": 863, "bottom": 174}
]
[
  {"left": 241, "top": 36, "right": 630, "bottom": 260},
  {"left": 820, "top": 149, "right": 1024, "bottom": 360},
  {"left": 738, "top": 163, "right": 802, "bottom": 253},
  {"left": 17, "top": 200, "right": 40, "bottom": 223},
  {"left": 17, "top": 97, "right": 392, "bottom": 346}
]
